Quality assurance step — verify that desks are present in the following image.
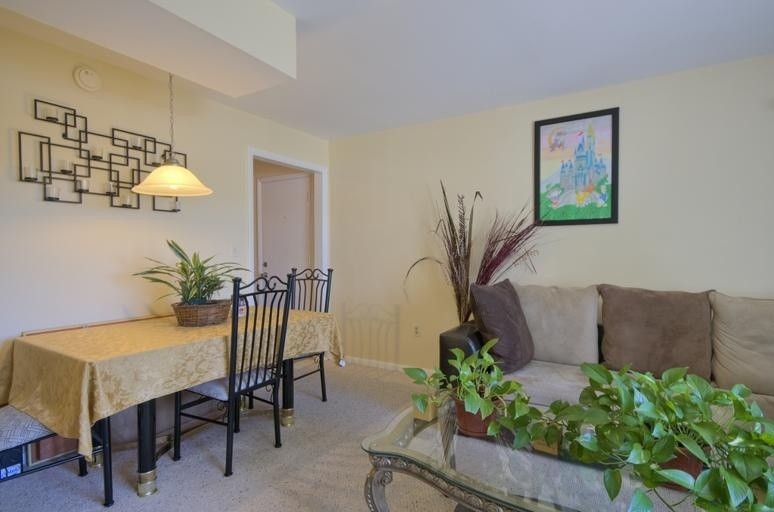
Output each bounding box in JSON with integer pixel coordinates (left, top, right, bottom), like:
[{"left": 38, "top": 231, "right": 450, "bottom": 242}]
[{"left": 21, "top": 310, "right": 330, "bottom": 507}]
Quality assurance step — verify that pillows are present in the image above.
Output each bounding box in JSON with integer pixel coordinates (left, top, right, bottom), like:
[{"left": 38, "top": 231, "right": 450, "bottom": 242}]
[{"left": 473, "top": 278, "right": 537, "bottom": 376}]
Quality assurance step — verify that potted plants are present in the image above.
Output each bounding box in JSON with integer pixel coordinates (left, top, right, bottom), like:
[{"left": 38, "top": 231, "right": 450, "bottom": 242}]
[{"left": 138, "top": 239, "right": 252, "bottom": 326}]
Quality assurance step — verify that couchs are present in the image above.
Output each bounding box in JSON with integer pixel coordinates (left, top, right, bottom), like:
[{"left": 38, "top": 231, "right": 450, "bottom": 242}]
[{"left": 437, "top": 278, "right": 774, "bottom": 409}]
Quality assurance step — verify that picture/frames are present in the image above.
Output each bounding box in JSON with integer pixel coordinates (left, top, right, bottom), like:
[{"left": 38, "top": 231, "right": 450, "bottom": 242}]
[{"left": 531, "top": 108, "right": 618, "bottom": 230}]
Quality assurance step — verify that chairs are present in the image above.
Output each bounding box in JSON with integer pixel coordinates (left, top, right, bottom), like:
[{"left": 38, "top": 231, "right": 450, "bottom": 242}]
[{"left": 177, "top": 267, "right": 333, "bottom": 476}]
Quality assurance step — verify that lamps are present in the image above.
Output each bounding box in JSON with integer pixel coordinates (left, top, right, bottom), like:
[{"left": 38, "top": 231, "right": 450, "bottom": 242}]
[{"left": 133, "top": 65, "right": 216, "bottom": 198}]
